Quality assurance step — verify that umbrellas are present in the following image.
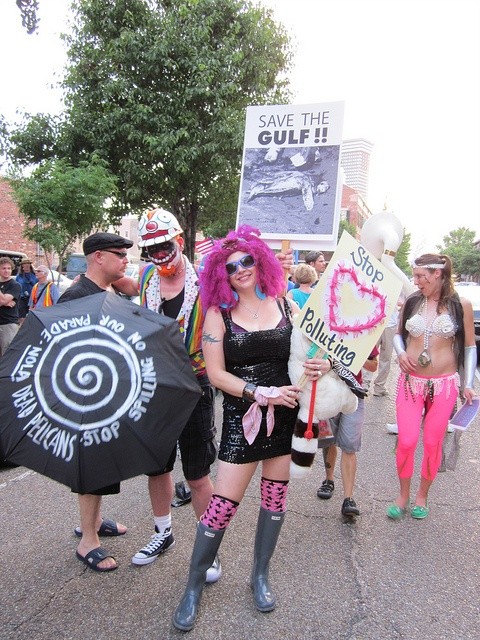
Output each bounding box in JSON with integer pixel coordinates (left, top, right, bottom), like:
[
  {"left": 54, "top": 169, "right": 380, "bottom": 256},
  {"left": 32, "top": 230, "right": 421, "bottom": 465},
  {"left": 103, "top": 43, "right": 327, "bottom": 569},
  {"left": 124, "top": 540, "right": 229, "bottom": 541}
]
[{"left": 0, "top": 292, "right": 206, "bottom": 496}]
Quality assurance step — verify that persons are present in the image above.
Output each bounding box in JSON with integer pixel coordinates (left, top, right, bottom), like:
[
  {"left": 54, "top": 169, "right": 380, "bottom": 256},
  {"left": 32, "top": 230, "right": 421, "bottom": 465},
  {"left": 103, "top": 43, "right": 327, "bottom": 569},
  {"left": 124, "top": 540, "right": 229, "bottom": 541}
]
[
  {"left": 317, "top": 343, "right": 380, "bottom": 517},
  {"left": 54, "top": 234, "right": 135, "bottom": 572},
  {"left": 71, "top": 208, "right": 292, "bottom": 583},
  {"left": 27, "top": 264, "right": 61, "bottom": 309},
  {"left": 286, "top": 263, "right": 319, "bottom": 309},
  {"left": 171, "top": 222, "right": 337, "bottom": 631},
  {"left": 304, "top": 249, "right": 327, "bottom": 276},
  {"left": 358, "top": 210, "right": 417, "bottom": 398},
  {"left": 0, "top": 255, "right": 22, "bottom": 355},
  {"left": 17, "top": 255, "right": 38, "bottom": 326},
  {"left": 386, "top": 255, "right": 477, "bottom": 520}
]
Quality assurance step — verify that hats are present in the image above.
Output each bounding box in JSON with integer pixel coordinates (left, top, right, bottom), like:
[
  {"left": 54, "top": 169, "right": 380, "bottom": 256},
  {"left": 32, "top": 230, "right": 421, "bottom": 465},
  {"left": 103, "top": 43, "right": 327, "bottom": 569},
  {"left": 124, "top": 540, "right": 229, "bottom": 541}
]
[
  {"left": 83, "top": 233, "right": 133, "bottom": 256},
  {"left": 19, "top": 258, "right": 33, "bottom": 264}
]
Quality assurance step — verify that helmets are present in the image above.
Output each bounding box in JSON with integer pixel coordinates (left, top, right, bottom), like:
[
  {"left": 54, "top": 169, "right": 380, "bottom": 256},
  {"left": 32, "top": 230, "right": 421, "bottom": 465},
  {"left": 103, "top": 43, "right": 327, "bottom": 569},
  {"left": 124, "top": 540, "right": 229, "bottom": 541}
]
[{"left": 138, "top": 209, "right": 184, "bottom": 247}]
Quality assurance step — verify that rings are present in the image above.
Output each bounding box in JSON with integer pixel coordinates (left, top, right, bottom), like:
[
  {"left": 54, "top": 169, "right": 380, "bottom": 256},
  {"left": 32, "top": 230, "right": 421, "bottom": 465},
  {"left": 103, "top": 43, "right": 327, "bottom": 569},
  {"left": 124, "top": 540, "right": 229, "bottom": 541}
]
[
  {"left": 315, "top": 371, "right": 323, "bottom": 377},
  {"left": 288, "top": 390, "right": 292, "bottom": 397}
]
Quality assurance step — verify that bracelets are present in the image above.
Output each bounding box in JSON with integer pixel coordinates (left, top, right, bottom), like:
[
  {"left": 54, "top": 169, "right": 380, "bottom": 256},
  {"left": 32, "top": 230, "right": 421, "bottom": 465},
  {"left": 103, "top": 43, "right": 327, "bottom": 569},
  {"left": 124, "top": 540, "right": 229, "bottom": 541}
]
[{"left": 242, "top": 382, "right": 258, "bottom": 404}]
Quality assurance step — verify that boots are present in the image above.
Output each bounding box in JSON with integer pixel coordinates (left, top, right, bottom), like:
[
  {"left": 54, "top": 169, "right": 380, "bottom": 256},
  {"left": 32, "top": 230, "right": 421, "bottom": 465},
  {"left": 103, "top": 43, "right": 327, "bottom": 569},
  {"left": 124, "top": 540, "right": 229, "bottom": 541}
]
[
  {"left": 250, "top": 507, "right": 285, "bottom": 612},
  {"left": 172, "top": 522, "right": 226, "bottom": 632}
]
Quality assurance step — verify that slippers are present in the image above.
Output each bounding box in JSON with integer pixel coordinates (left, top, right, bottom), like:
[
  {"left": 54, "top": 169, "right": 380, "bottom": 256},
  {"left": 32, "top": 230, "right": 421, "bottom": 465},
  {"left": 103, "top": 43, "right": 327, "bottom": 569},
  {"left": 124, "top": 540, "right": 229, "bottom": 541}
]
[
  {"left": 74, "top": 519, "right": 127, "bottom": 536},
  {"left": 76, "top": 546, "right": 118, "bottom": 572}
]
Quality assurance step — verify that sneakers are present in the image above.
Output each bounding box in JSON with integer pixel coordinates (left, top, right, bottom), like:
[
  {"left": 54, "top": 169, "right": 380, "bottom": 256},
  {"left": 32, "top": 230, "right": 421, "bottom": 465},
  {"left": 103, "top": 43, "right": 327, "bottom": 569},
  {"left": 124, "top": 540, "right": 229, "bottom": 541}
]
[
  {"left": 342, "top": 497, "right": 359, "bottom": 516},
  {"left": 447, "top": 420, "right": 454, "bottom": 432},
  {"left": 387, "top": 423, "right": 398, "bottom": 434},
  {"left": 411, "top": 496, "right": 430, "bottom": 519},
  {"left": 206, "top": 551, "right": 222, "bottom": 582},
  {"left": 317, "top": 480, "right": 334, "bottom": 499},
  {"left": 387, "top": 499, "right": 410, "bottom": 517},
  {"left": 132, "top": 525, "right": 175, "bottom": 564}
]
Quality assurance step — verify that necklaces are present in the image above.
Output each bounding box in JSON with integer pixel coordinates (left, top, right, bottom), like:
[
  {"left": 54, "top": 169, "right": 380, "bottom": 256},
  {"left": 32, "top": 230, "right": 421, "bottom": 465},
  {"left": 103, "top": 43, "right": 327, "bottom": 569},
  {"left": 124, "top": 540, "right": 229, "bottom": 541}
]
[
  {"left": 417, "top": 297, "right": 440, "bottom": 368},
  {"left": 235, "top": 298, "right": 266, "bottom": 319}
]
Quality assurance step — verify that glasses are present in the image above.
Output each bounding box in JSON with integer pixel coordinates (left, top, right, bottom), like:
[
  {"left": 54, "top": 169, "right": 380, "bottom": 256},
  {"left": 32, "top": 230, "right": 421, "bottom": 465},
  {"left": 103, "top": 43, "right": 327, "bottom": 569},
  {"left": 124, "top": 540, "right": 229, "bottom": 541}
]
[
  {"left": 35, "top": 270, "right": 44, "bottom": 272},
  {"left": 104, "top": 250, "right": 128, "bottom": 258},
  {"left": 225, "top": 254, "right": 256, "bottom": 276}
]
